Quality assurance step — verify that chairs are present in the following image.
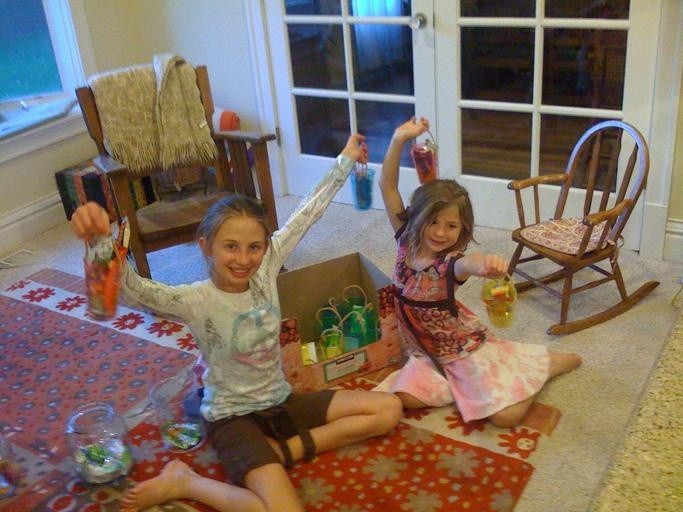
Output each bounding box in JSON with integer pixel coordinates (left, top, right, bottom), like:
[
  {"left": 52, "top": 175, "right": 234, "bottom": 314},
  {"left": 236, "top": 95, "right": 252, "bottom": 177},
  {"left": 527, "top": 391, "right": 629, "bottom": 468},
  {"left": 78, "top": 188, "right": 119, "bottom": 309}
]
[
  {"left": 468, "top": 33, "right": 534, "bottom": 102},
  {"left": 547, "top": 27, "right": 602, "bottom": 106},
  {"left": 504, "top": 119, "right": 660, "bottom": 336},
  {"left": 74, "top": 65, "right": 276, "bottom": 280}
]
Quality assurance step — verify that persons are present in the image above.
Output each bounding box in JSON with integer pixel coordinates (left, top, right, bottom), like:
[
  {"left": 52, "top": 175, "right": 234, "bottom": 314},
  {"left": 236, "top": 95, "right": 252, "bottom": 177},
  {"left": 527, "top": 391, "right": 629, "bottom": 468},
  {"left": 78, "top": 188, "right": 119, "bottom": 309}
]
[
  {"left": 71, "top": 134, "right": 402, "bottom": 512},
  {"left": 369, "top": 116, "right": 584, "bottom": 428}
]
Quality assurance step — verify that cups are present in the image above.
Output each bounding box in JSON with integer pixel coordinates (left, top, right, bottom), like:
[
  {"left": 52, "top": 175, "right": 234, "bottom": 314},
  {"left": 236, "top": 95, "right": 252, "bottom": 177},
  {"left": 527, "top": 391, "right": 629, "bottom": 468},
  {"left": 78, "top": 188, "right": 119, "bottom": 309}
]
[
  {"left": 482, "top": 282, "right": 517, "bottom": 325},
  {"left": 148, "top": 367, "right": 207, "bottom": 452},
  {"left": 352, "top": 170, "right": 376, "bottom": 209},
  {"left": 85, "top": 249, "right": 126, "bottom": 318},
  {"left": 297, "top": 296, "right": 380, "bottom": 365},
  {"left": 410, "top": 140, "right": 439, "bottom": 185}
]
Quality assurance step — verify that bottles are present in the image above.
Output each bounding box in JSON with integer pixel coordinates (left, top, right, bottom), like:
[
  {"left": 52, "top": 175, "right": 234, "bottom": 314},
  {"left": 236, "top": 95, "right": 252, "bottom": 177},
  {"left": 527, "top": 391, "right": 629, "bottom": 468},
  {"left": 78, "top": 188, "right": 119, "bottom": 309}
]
[{"left": 67, "top": 401, "right": 134, "bottom": 485}]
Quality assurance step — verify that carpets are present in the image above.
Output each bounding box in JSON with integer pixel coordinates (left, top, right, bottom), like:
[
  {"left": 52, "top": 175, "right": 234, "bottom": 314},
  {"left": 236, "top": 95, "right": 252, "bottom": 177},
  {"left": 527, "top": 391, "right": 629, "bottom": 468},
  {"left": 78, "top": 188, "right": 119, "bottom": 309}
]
[{"left": 1, "top": 195, "right": 682, "bottom": 512}]
